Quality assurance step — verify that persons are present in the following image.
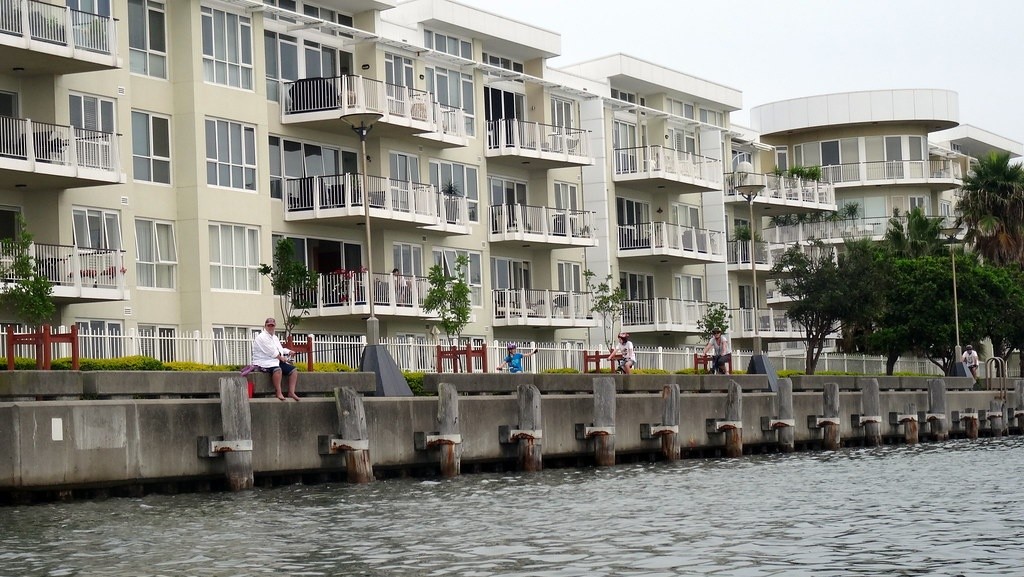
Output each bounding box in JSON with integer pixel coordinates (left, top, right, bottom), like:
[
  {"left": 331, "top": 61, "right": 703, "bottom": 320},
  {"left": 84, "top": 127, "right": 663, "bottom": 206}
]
[
  {"left": 963, "top": 345, "right": 979, "bottom": 379},
  {"left": 702, "top": 327, "right": 731, "bottom": 374},
  {"left": 253, "top": 318, "right": 301, "bottom": 401},
  {"left": 393, "top": 268, "right": 407, "bottom": 307},
  {"left": 608, "top": 333, "right": 636, "bottom": 374},
  {"left": 497, "top": 344, "right": 538, "bottom": 373}
]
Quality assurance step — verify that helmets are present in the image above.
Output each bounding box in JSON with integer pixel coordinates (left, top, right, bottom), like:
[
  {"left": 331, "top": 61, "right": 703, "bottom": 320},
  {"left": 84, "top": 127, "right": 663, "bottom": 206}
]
[
  {"left": 966, "top": 345, "right": 972, "bottom": 349},
  {"left": 618, "top": 332, "right": 629, "bottom": 339},
  {"left": 712, "top": 327, "right": 722, "bottom": 331},
  {"left": 507, "top": 344, "right": 516, "bottom": 349}
]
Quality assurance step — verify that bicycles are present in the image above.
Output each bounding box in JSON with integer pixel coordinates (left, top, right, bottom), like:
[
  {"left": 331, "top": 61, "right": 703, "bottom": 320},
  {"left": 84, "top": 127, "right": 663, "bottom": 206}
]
[
  {"left": 700, "top": 353, "right": 730, "bottom": 375},
  {"left": 607, "top": 357, "right": 632, "bottom": 374}
]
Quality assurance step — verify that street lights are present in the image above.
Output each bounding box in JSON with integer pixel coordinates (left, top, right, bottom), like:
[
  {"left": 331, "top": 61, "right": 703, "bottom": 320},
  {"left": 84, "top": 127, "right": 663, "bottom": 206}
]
[
  {"left": 735, "top": 185, "right": 780, "bottom": 392},
  {"left": 338, "top": 112, "right": 413, "bottom": 398},
  {"left": 940, "top": 228, "right": 973, "bottom": 377}
]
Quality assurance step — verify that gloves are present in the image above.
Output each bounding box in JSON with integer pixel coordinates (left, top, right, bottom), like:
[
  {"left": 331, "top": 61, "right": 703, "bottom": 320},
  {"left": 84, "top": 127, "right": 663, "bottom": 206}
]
[{"left": 534, "top": 349, "right": 538, "bottom": 354}]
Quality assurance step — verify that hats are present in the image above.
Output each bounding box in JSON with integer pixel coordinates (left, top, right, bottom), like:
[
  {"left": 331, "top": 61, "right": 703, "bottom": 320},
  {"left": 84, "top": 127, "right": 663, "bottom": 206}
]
[{"left": 265, "top": 318, "right": 276, "bottom": 326}]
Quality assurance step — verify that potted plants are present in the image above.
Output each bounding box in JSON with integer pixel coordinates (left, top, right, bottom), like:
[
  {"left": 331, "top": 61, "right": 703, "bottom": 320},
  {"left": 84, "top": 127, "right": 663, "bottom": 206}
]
[{"left": 768, "top": 202, "right": 859, "bottom": 243}]
